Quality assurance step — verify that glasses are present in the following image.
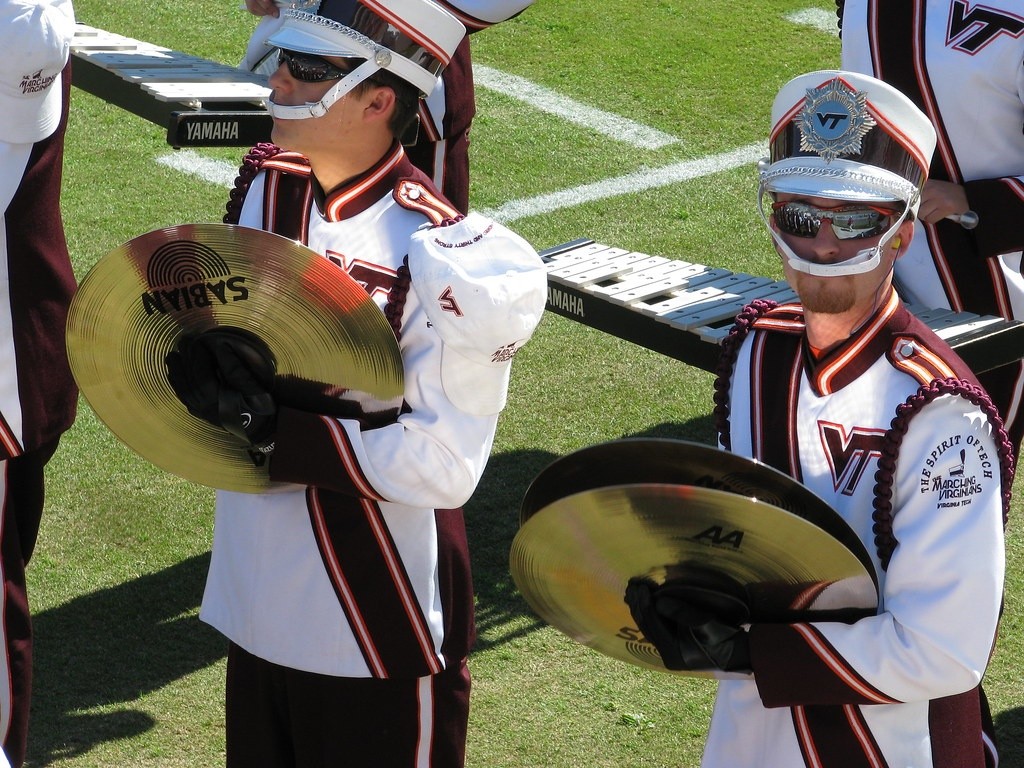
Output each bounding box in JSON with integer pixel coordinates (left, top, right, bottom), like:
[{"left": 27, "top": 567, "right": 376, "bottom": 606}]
[
  {"left": 277, "top": 46, "right": 411, "bottom": 108},
  {"left": 771, "top": 200, "right": 914, "bottom": 241}
]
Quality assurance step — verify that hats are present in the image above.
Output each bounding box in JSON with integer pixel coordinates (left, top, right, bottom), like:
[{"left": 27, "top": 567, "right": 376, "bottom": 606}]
[
  {"left": 263, "top": 0, "right": 466, "bottom": 119},
  {"left": 756, "top": 70, "right": 937, "bottom": 276},
  {"left": 0, "top": 0, "right": 75, "bottom": 144}
]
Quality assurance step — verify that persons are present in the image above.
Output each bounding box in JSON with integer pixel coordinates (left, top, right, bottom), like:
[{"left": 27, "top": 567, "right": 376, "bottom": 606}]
[
  {"left": 624, "top": 70, "right": 1016, "bottom": 767},
  {"left": 836, "top": 0, "right": 1024, "bottom": 458},
  {"left": 1, "top": 0, "right": 80, "bottom": 768},
  {"left": 164, "top": 0, "right": 551, "bottom": 768},
  {"left": 238, "top": 0, "right": 532, "bottom": 219}
]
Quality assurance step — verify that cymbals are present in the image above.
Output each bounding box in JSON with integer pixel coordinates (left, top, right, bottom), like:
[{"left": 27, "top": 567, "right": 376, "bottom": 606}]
[
  {"left": 508, "top": 437, "right": 881, "bottom": 680},
  {"left": 63, "top": 224, "right": 406, "bottom": 495}
]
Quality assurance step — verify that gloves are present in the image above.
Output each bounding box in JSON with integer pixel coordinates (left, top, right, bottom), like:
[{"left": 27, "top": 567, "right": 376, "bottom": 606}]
[
  {"left": 165, "top": 333, "right": 279, "bottom": 445},
  {"left": 625, "top": 578, "right": 758, "bottom": 675}
]
